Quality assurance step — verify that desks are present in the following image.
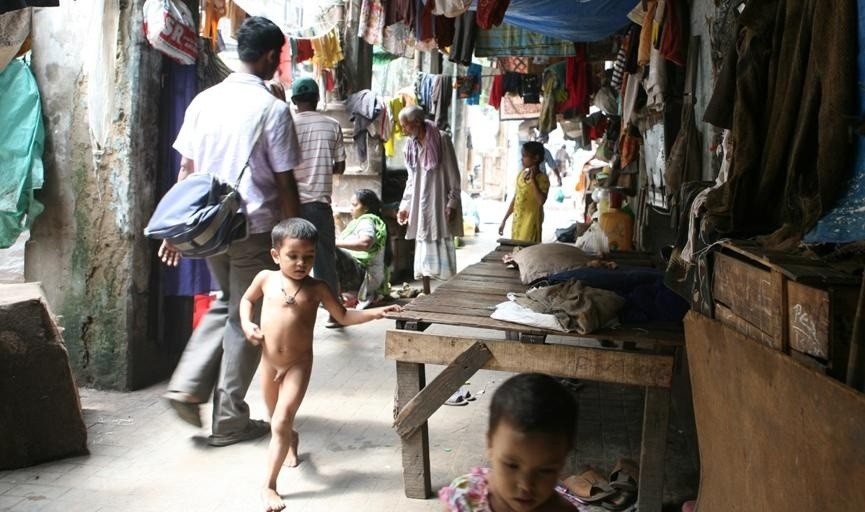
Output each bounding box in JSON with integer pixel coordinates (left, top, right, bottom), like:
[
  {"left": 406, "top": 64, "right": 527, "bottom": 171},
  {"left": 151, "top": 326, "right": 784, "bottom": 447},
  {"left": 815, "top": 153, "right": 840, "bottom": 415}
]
[{"left": 383, "top": 236, "right": 684, "bottom": 512}]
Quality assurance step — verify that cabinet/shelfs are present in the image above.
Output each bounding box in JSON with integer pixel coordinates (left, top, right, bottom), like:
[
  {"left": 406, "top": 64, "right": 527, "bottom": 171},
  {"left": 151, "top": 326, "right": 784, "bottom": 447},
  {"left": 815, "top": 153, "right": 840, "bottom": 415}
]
[
  {"left": 315, "top": 102, "right": 383, "bottom": 242},
  {"left": 478, "top": 147, "right": 507, "bottom": 201}
]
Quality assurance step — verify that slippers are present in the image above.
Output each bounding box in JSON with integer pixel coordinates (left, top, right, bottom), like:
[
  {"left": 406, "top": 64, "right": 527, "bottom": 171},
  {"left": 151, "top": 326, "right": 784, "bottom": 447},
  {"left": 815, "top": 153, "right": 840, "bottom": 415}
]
[
  {"left": 444, "top": 390, "right": 474, "bottom": 405},
  {"left": 561, "top": 460, "right": 638, "bottom": 510}
]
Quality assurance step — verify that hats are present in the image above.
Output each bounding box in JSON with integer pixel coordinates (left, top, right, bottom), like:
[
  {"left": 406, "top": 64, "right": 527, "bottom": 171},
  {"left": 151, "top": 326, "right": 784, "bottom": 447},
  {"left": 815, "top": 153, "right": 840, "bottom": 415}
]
[{"left": 292, "top": 77, "right": 319, "bottom": 95}]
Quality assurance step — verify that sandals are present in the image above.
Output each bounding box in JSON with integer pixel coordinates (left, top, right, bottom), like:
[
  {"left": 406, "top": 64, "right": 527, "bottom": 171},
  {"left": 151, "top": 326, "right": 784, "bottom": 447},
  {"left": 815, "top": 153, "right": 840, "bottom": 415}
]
[
  {"left": 209, "top": 418, "right": 269, "bottom": 445},
  {"left": 169, "top": 394, "right": 201, "bottom": 427}
]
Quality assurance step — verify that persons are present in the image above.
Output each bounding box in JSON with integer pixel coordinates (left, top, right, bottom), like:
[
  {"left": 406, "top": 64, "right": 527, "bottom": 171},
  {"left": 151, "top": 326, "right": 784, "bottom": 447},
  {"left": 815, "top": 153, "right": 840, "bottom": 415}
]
[
  {"left": 498, "top": 142, "right": 550, "bottom": 243},
  {"left": 557, "top": 145, "right": 570, "bottom": 172},
  {"left": 335, "top": 189, "right": 390, "bottom": 308},
  {"left": 435, "top": 373, "right": 584, "bottom": 512},
  {"left": 239, "top": 217, "right": 403, "bottom": 511},
  {"left": 540, "top": 149, "right": 563, "bottom": 187},
  {"left": 156, "top": 17, "right": 298, "bottom": 444},
  {"left": 289, "top": 77, "right": 346, "bottom": 327},
  {"left": 395, "top": 106, "right": 463, "bottom": 293}
]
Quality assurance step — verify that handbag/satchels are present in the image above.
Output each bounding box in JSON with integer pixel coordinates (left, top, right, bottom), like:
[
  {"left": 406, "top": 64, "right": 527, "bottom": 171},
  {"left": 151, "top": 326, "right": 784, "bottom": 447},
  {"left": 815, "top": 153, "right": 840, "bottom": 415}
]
[
  {"left": 142, "top": 0, "right": 198, "bottom": 65},
  {"left": 144, "top": 173, "right": 249, "bottom": 260}
]
